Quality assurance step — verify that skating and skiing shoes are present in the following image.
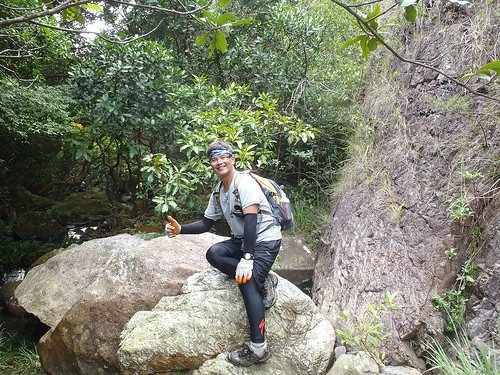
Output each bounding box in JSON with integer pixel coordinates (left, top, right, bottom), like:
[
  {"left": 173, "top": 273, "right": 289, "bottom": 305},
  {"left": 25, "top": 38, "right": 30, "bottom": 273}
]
[{"left": 227, "top": 343, "right": 270, "bottom": 367}]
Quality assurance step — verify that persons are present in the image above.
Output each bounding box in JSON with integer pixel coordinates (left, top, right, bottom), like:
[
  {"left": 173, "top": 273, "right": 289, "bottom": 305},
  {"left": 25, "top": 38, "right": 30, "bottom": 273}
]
[{"left": 165, "top": 143, "right": 284, "bottom": 367}]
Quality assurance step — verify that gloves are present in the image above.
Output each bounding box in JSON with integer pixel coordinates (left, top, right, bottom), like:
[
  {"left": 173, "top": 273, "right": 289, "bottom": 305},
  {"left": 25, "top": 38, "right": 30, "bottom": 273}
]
[
  {"left": 235, "top": 258, "right": 254, "bottom": 284},
  {"left": 165, "top": 216, "right": 181, "bottom": 238}
]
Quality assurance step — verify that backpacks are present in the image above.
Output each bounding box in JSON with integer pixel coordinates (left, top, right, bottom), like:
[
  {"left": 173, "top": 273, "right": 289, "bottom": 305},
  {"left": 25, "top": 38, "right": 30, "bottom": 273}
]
[{"left": 213, "top": 173, "right": 293, "bottom": 230}]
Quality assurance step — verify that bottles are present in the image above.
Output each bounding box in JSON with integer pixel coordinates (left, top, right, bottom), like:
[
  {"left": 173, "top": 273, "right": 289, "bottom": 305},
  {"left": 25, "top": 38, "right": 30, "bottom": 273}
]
[{"left": 281, "top": 193, "right": 289, "bottom": 202}]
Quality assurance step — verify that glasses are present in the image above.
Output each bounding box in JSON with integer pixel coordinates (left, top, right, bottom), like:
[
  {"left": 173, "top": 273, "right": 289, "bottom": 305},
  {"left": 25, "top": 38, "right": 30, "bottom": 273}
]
[{"left": 211, "top": 156, "right": 228, "bottom": 161}]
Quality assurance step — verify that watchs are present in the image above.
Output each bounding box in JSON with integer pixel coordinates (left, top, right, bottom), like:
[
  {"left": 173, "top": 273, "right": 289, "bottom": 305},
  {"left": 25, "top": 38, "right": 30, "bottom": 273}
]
[{"left": 242, "top": 253, "right": 254, "bottom": 260}]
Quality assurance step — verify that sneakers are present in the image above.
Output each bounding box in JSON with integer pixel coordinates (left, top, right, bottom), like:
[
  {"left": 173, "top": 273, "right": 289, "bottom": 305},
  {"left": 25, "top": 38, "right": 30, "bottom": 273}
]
[{"left": 262, "top": 274, "right": 278, "bottom": 309}]
